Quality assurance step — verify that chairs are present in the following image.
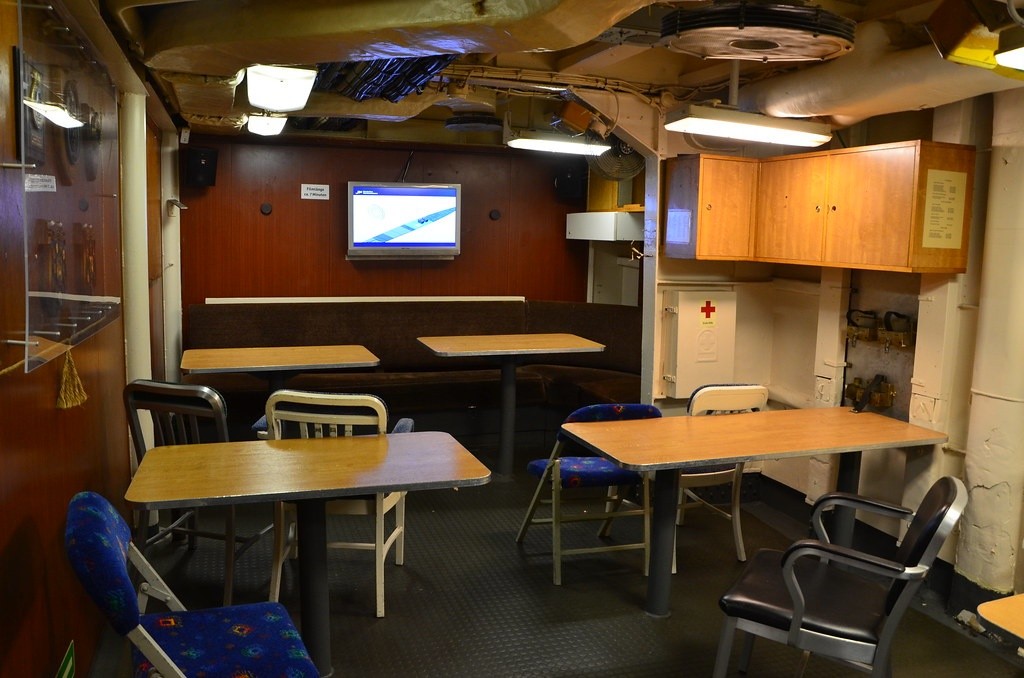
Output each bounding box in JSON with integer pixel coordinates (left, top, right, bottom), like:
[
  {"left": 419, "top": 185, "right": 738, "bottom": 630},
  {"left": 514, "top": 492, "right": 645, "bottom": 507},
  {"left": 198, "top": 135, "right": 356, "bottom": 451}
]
[
  {"left": 263, "top": 391, "right": 404, "bottom": 618},
  {"left": 124, "top": 377, "right": 275, "bottom": 610},
  {"left": 516, "top": 400, "right": 662, "bottom": 586},
  {"left": 712, "top": 475, "right": 968, "bottom": 678},
  {"left": 65, "top": 489, "right": 318, "bottom": 678},
  {"left": 603, "top": 382, "right": 770, "bottom": 574}
]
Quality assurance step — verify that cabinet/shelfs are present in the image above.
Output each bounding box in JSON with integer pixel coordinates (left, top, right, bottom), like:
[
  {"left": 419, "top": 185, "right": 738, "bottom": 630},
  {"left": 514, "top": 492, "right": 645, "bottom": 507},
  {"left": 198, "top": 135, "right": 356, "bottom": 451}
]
[{"left": 661, "top": 139, "right": 975, "bottom": 275}]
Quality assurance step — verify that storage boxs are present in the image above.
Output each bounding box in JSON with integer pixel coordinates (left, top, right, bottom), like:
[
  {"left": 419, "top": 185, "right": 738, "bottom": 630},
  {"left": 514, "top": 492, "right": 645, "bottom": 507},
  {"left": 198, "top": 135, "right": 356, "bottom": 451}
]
[{"left": 587, "top": 164, "right": 635, "bottom": 212}]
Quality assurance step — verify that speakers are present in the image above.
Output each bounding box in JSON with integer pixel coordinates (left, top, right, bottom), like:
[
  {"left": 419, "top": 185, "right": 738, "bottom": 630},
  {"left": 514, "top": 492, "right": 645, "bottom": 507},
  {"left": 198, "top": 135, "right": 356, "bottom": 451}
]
[
  {"left": 553, "top": 156, "right": 588, "bottom": 206},
  {"left": 181, "top": 145, "right": 219, "bottom": 187}
]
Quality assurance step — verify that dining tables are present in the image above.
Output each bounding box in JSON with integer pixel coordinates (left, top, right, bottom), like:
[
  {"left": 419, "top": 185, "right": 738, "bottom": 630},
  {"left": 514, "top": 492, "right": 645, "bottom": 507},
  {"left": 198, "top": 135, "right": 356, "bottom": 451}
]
[
  {"left": 560, "top": 404, "right": 948, "bottom": 617},
  {"left": 181, "top": 344, "right": 381, "bottom": 440},
  {"left": 125, "top": 431, "right": 492, "bottom": 677},
  {"left": 955, "top": 593, "right": 1024, "bottom": 678},
  {"left": 417, "top": 332, "right": 606, "bottom": 477}
]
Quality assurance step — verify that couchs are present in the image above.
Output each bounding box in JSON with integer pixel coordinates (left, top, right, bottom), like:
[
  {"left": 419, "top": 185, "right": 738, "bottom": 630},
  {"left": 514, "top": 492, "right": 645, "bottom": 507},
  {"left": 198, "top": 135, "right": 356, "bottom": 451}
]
[{"left": 181, "top": 302, "right": 642, "bottom": 445}]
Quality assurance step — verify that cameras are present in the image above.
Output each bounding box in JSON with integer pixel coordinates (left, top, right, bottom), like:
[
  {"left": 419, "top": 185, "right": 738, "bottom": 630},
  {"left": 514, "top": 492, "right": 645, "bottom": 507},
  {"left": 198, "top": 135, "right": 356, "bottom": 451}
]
[{"left": 346, "top": 180, "right": 462, "bottom": 257}]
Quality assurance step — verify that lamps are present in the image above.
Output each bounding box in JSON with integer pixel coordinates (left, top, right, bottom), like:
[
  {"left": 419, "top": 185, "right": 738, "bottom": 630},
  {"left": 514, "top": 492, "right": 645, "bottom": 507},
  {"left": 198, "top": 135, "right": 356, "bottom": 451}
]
[
  {"left": 244, "top": 62, "right": 319, "bottom": 112},
  {"left": 662, "top": 59, "right": 833, "bottom": 147},
  {"left": 23, "top": 63, "right": 84, "bottom": 129},
  {"left": 994, "top": 25, "right": 1024, "bottom": 72},
  {"left": 502, "top": 109, "right": 612, "bottom": 156},
  {"left": 247, "top": 115, "right": 288, "bottom": 136}
]
